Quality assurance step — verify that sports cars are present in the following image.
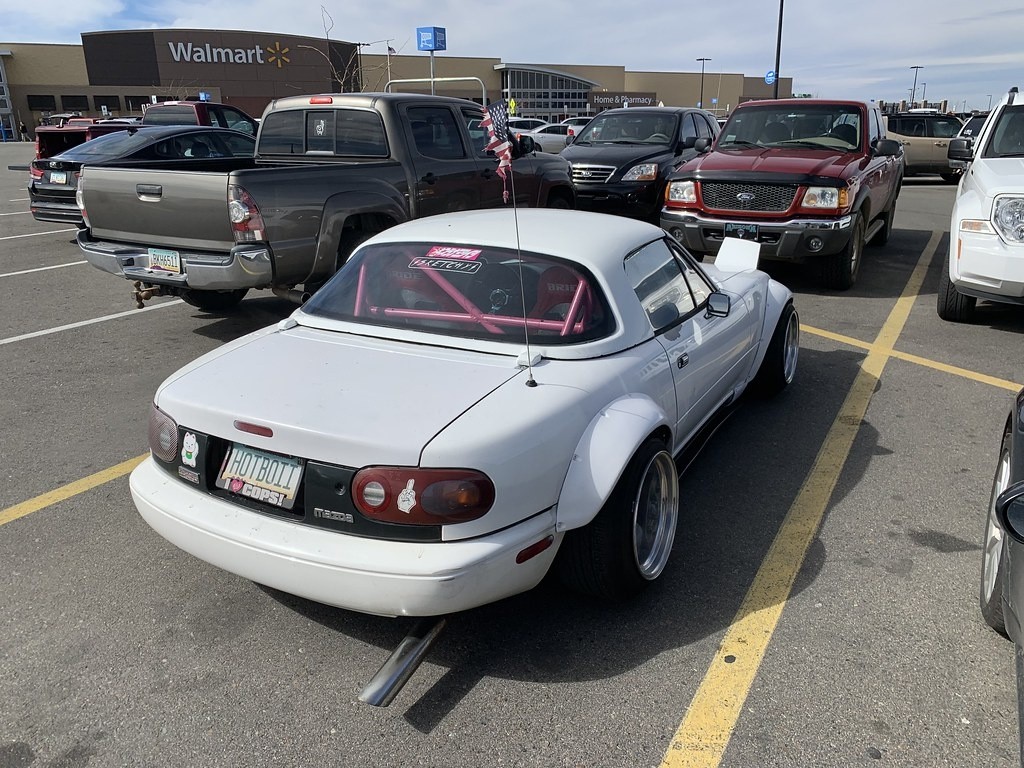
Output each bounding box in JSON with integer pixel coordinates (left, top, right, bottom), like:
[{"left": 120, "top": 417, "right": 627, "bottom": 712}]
[{"left": 128, "top": 206, "right": 800, "bottom": 708}]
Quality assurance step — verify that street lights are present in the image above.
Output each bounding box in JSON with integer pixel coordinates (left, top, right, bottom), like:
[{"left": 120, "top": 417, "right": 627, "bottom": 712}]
[
  {"left": 911, "top": 66, "right": 924, "bottom": 109},
  {"left": 696, "top": 58, "right": 711, "bottom": 109},
  {"left": 987, "top": 95, "right": 992, "bottom": 111},
  {"left": 963, "top": 100, "right": 966, "bottom": 113}
]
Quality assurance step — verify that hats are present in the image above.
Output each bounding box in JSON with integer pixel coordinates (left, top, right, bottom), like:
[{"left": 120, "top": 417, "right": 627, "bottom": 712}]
[{"left": 777, "top": 114, "right": 798, "bottom": 123}]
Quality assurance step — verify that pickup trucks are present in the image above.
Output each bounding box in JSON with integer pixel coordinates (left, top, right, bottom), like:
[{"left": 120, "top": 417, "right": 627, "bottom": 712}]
[
  {"left": 8, "top": 101, "right": 261, "bottom": 172},
  {"left": 660, "top": 97, "right": 906, "bottom": 291},
  {"left": 74, "top": 92, "right": 579, "bottom": 312}
]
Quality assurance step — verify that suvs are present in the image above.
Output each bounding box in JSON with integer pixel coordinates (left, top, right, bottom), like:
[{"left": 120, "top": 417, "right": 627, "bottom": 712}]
[
  {"left": 557, "top": 106, "right": 721, "bottom": 227},
  {"left": 937, "top": 87, "right": 1024, "bottom": 324},
  {"left": 880, "top": 107, "right": 967, "bottom": 185},
  {"left": 947, "top": 111, "right": 994, "bottom": 177}
]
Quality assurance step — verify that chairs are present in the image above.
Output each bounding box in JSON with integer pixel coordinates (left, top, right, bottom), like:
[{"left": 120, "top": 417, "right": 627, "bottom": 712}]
[
  {"left": 758, "top": 121, "right": 790, "bottom": 145},
  {"left": 191, "top": 142, "right": 211, "bottom": 159},
  {"left": 828, "top": 123, "right": 857, "bottom": 149}
]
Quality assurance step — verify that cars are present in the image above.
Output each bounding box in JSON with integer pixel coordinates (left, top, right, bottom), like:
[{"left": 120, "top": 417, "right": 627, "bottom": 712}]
[
  {"left": 469, "top": 116, "right": 606, "bottom": 155},
  {"left": 42, "top": 112, "right": 262, "bottom": 156},
  {"left": 979, "top": 385, "right": 1024, "bottom": 768},
  {"left": 27, "top": 125, "right": 259, "bottom": 227}
]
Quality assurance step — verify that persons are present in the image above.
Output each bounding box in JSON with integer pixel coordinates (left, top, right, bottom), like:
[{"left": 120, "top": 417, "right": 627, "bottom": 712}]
[{"left": 19, "top": 121, "right": 32, "bottom": 142}]
